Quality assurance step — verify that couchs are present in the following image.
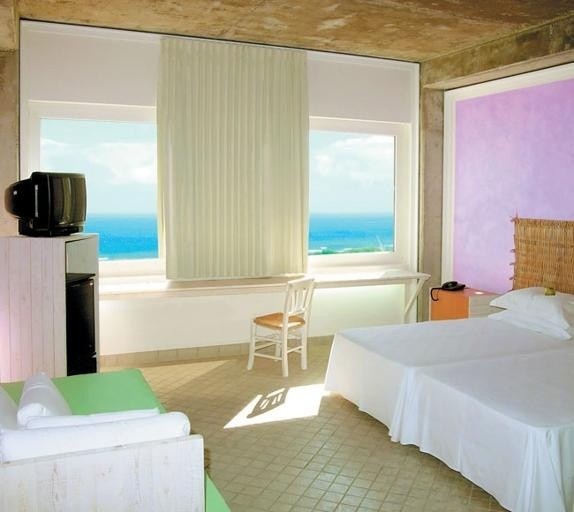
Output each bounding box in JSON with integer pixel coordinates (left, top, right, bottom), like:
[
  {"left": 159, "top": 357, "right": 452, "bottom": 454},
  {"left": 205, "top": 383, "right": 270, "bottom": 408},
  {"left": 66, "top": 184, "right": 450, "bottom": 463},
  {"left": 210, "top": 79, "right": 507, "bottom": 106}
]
[{"left": 0, "top": 383, "right": 205, "bottom": 512}]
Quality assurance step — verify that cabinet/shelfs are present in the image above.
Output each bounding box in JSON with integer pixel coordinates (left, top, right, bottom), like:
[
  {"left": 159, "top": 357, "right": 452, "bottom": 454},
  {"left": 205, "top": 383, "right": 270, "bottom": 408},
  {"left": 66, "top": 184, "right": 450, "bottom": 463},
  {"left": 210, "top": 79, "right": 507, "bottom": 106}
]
[{"left": 2, "top": 235, "right": 100, "bottom": 379}]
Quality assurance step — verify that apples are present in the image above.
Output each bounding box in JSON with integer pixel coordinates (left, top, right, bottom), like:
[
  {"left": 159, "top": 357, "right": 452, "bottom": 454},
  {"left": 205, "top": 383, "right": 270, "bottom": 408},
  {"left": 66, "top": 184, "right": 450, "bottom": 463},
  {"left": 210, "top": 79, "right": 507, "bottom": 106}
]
[{"left": 545, "top": 287, "right": 555, "bottom": 295}]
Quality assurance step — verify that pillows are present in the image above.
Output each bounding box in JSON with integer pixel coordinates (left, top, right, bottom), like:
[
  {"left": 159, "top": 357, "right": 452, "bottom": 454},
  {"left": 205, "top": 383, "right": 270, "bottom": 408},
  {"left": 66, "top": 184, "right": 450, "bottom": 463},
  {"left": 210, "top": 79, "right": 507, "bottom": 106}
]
[
  {"left": 489, "top": 287, "right": 574, "bottom": 334},
  {"left": 0, "top": 371, "right": 190, "bottom": 463},
  {"left": 488, "top": 309, "right": 574, "bottom": 342}
]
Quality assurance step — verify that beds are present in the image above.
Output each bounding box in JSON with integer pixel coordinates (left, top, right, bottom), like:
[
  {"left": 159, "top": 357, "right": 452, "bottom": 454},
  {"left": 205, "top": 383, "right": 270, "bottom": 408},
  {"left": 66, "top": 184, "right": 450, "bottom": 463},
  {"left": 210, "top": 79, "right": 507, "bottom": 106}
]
[{"left": 323, "top": 218, "right": 574, "bottom": 512}]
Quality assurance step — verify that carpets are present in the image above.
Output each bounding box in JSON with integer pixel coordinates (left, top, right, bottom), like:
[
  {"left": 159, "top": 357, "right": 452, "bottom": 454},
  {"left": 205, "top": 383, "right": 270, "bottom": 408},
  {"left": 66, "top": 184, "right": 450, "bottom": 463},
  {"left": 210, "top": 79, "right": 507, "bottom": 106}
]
[{"left": 0, "top": 367, "right": 231, "bottom": 512}]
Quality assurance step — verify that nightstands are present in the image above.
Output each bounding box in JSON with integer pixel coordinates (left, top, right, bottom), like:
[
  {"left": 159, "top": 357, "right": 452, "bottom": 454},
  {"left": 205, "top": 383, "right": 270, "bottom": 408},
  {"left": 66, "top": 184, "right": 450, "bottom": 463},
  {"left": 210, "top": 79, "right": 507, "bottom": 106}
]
[{"left": 430, "top": 286, "right": 505, "bottom": 321}]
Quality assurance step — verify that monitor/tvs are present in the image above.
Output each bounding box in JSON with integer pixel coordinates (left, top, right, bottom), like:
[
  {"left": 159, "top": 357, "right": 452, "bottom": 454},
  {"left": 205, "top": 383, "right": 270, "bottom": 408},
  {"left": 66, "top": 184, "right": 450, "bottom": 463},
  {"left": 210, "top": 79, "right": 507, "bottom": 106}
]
[{"left": 5, "top": 171, "right": 86, "bottom": 235}]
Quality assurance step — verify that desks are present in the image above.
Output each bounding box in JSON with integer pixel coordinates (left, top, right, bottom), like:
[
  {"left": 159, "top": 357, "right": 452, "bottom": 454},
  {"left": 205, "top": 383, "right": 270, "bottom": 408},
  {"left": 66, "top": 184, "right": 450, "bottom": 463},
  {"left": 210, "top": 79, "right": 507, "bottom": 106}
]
[{"left": 98, "top": 271, "right": 432, "bottom": 322}]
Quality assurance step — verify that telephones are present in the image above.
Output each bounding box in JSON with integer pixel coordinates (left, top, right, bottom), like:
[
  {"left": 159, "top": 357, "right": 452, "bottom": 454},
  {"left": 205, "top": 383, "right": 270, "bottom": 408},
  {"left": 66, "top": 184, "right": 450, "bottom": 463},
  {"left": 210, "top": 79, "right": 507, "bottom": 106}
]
[{"left": 443, "top": 281, "right": 466, "bottom": 291}]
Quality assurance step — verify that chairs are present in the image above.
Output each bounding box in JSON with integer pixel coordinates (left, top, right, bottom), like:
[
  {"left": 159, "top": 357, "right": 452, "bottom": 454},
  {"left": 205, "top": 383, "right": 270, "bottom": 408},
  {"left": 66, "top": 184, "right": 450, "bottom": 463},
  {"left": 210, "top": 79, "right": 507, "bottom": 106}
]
[{"left": 246, "top": 277, "right": 316, "bottom": 378}]
